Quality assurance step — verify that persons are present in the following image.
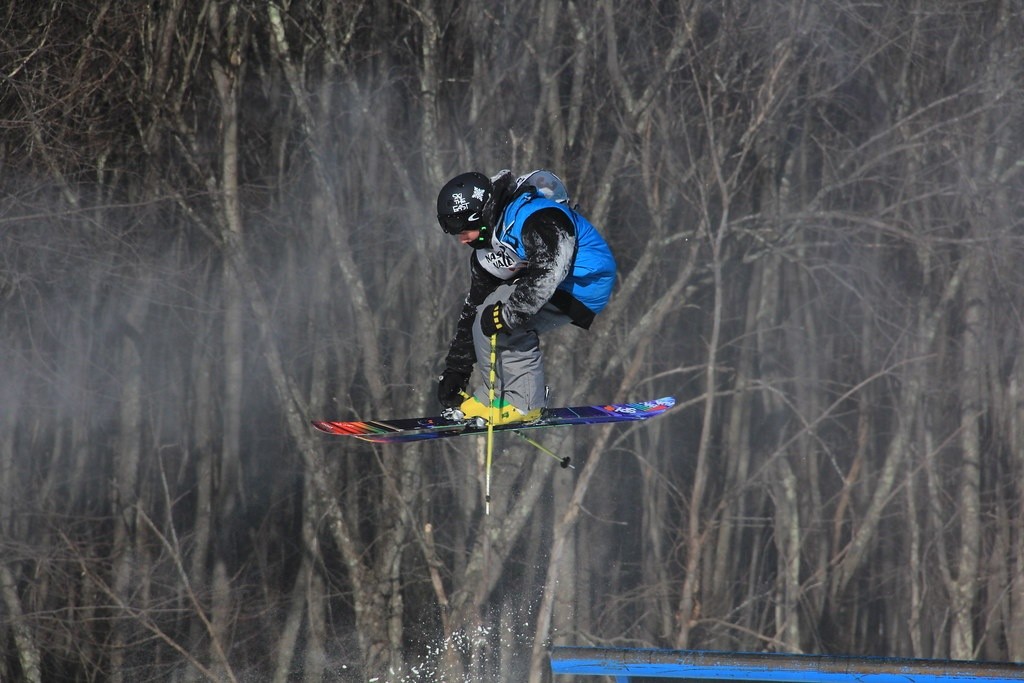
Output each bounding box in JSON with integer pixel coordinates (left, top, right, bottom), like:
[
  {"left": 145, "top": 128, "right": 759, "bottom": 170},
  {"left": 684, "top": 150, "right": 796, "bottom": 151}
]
[{"left": 436, "top": 168, "right": 616, "bottom": 426}]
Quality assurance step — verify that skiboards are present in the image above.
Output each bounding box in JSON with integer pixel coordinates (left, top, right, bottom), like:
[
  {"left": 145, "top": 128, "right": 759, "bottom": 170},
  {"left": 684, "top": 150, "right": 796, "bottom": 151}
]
[{"left": 310, "top": 397, "right": 675, "bottom": 443}]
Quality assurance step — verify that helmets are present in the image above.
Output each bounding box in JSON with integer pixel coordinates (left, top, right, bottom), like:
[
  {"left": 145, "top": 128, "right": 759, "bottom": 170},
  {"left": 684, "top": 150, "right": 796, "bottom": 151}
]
[{"left": 436, "top": 171, "right": 492, "bottom": 236}]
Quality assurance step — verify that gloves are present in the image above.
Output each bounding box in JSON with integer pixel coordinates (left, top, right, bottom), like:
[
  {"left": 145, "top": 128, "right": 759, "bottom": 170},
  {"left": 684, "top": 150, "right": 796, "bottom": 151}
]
[
  {"left": 480, "top": 303, "right": 511, "bottom": 338},
  {"left": 437, "top": 368, "right": 471, "bottom": 409}
]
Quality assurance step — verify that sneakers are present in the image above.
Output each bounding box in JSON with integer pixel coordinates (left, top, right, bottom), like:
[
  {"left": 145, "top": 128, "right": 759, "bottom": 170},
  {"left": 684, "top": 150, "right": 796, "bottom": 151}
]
[
  {"left": 474, "top": 398, "right": 541, "bottom": 426},
  {"left": 450, "top": 395, "right": 494, "bottom": 421}
]
